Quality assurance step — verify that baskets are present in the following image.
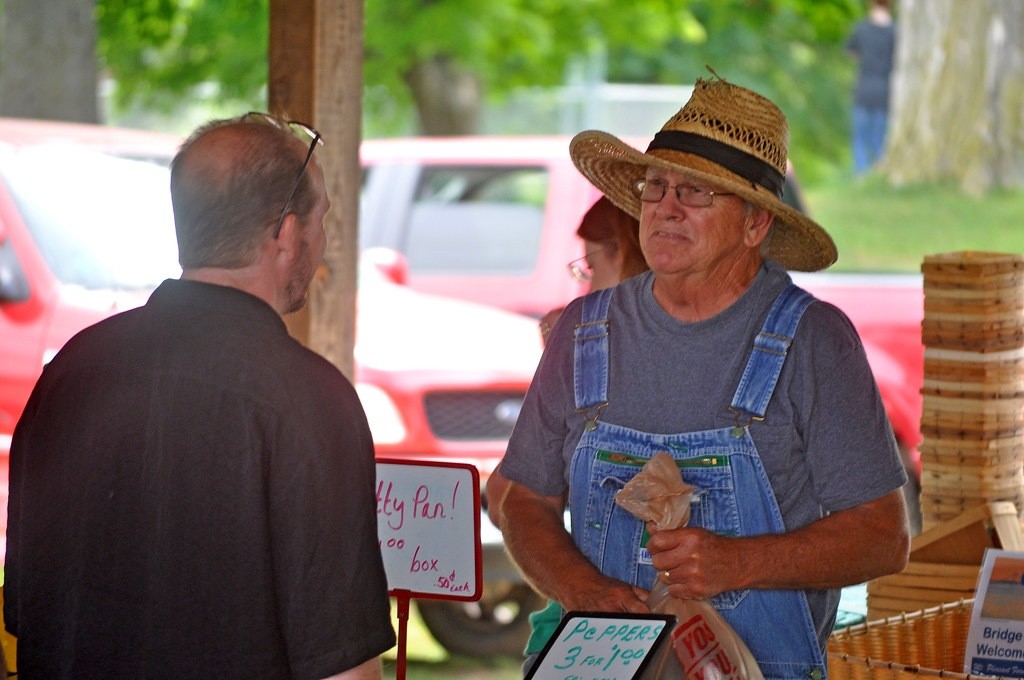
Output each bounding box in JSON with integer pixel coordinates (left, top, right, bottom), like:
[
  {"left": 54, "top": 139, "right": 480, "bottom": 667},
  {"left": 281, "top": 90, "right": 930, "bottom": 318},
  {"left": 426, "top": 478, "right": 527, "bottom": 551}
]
[{"left": 824, "top": 598, "right": 994, "bottom": 680}]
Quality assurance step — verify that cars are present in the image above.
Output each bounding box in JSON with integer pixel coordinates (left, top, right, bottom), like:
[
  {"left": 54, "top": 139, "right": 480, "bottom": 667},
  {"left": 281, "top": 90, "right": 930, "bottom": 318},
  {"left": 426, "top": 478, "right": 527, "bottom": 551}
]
[
  {"left": 0, "top": 115, "right": 574, "bottom": 659},
  {"left": 357, "top": 129, "right": 924, "bottom": 545}
]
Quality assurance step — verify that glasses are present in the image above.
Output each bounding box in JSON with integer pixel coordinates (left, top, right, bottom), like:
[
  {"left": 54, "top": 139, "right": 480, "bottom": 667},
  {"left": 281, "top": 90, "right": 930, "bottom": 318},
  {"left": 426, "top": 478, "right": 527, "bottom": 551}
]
[
  {"left": 631, "top": 177, "right": 736, "bottom": 208},
  {"left": 568, "top": 246, "right": 610, "bottom": 281},
  {"left": 238, "top": 110, "right": 325, "bottom": 239}
]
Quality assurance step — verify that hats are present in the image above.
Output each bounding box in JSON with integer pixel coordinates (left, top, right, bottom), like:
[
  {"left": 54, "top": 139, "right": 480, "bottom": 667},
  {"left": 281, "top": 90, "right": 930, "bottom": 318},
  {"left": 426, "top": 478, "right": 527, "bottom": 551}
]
[{"left": 567, "top": 66, "right": 839, "bottom": 272}]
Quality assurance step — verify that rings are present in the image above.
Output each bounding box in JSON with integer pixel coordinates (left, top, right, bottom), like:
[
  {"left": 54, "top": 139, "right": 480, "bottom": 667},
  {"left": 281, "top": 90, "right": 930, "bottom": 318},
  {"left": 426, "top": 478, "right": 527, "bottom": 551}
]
[{"left": 665, "top": 570, "right": 672, "bottom": 581}]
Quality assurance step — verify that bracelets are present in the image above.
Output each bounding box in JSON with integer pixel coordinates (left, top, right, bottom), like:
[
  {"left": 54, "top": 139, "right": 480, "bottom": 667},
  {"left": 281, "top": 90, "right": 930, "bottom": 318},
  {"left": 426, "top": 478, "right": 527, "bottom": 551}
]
[{"left": 541, "top": 321, "right": 550, "bottom": 336}]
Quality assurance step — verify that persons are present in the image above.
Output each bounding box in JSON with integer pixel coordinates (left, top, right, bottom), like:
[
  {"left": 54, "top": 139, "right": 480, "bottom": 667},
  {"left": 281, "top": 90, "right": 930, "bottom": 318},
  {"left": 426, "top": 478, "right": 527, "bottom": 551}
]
[
  {"left": 485, "top": 78, "right": 909, "bottom": 680},
  {"left": 4, "top": 110, "right": 397, "bottom": 680},
  {"left": 486, "top": 193, "right": 653, "bottom": 530}
]
